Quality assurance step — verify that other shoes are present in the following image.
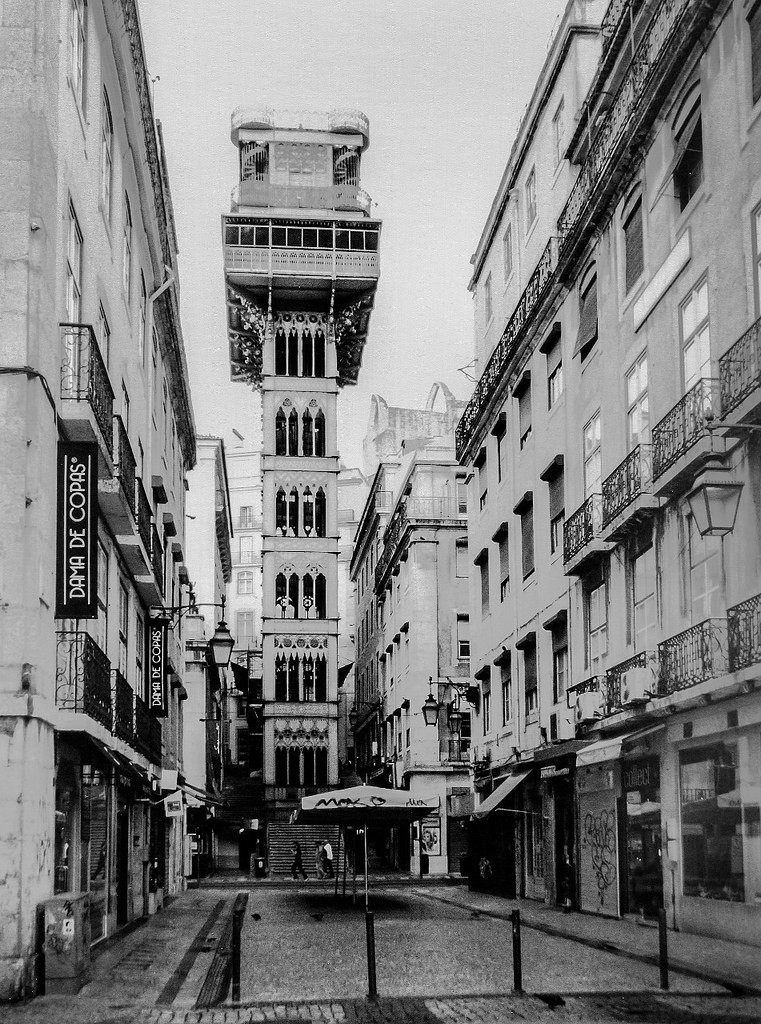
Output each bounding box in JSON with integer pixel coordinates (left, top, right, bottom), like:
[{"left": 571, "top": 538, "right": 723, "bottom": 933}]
[
  {"left": 328, "top": 875, "right": 336, "bottom": 879},
  {"left": 304, "top": 878, "right": 309, "bottom": 881},
  {"left": 323, "top": 873, "right": 327, "bottom": 879},
  {"left": 292, "top": 878, "right": 298, "bottom": 880},
  {"left": 316, "top": 875, "right": 322, "bottom": 878}
]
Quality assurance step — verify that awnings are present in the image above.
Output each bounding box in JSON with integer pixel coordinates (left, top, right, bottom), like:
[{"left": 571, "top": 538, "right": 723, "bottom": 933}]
[
  {"left": 576, "top": 721, "right": 666, "bottom": 765},
  {"left": 469, "top": 763, "right": 534, "bottom": 812}
]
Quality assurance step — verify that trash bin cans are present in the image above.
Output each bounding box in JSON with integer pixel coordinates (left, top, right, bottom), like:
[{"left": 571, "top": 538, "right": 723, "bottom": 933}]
[
  {"left": 255, "top": 857, "right": 264, "bottom": 878},
  {"left": 458, "top": 855, "right": 468, "bottom": 877}
]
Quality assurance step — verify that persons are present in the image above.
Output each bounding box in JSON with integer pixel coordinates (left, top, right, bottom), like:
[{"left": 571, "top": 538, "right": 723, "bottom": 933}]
[
  {"left": 314, "top": 839, "right": 335, "bottom": 880},
  {"left": 289, "top": 841, "right": 309, "bottom": 882},
  {"left": 422, "top": 830, "right": 434, "bottom": 855}
]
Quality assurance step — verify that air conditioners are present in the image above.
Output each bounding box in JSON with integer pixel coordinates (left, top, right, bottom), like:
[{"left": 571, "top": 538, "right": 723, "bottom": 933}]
[
  {"left": 621, "top": 668, "right": 652, "bottom": 705},
  {"left": 576, "top": 692, "right": 600, "bottom": 722},
  {"left": 469, "top": 745, "right": 486, "bottom": 763}
]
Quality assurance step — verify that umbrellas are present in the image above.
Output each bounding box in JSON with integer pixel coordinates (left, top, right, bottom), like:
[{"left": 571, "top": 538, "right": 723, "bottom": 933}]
[{"left": 301, "top": 783, "right": 440, "bottom": 906}]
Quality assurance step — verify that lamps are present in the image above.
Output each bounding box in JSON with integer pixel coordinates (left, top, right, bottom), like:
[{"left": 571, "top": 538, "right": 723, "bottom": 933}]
[
  {"left": 349, "top": 699, "right": 380, "bottom": 726},
  {"left": 686, "top": 413, "right": 761, "bottom": 540},
  {"left": 421, "top": 675, "right": 480, "bottom": 733},
  {"left": 151, "top": 595, "right": 235, "bottom": 666}
]
